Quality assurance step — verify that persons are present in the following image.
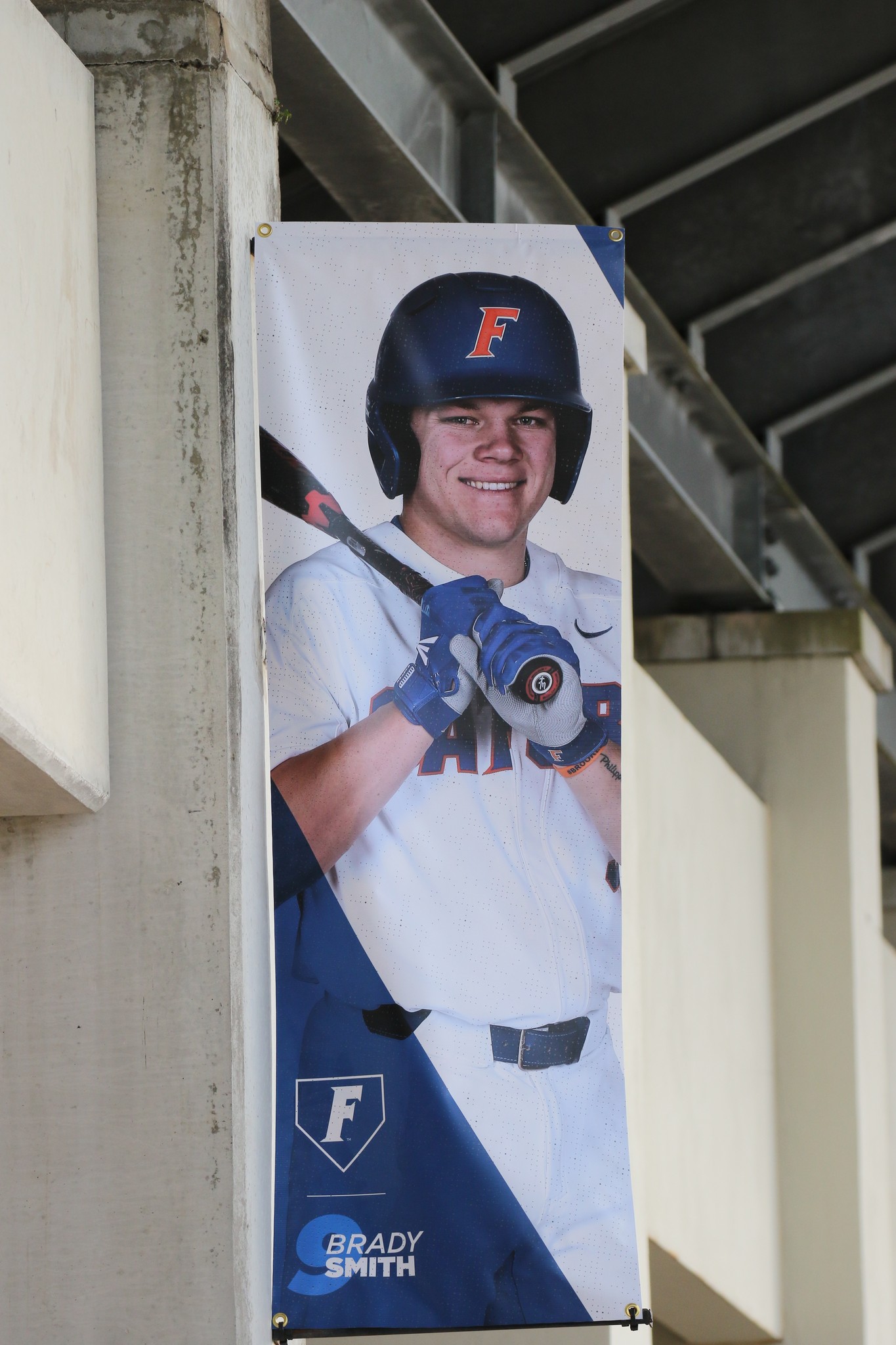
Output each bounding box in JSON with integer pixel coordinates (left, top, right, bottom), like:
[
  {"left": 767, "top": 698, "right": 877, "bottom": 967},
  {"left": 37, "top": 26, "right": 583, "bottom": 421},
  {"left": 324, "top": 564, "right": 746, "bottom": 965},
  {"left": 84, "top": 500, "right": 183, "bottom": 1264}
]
[{"left": 256, "top": 271, "right": 646, "bottom": 1327}]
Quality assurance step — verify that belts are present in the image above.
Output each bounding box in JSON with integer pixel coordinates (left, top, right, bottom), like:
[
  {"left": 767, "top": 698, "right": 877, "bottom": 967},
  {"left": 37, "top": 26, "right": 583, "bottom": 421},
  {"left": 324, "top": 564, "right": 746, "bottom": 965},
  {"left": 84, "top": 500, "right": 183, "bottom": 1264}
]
[{"left": 491, "top": 1016, "right": 590, "bottom": 1070}]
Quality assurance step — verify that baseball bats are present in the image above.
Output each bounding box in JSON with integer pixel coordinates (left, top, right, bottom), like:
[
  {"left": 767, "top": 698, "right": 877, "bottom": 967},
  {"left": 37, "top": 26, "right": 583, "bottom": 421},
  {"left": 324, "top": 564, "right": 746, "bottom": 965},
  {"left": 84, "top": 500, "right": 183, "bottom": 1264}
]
[{"left": 258, "top": 424, "right": 567, "bottom": 705}]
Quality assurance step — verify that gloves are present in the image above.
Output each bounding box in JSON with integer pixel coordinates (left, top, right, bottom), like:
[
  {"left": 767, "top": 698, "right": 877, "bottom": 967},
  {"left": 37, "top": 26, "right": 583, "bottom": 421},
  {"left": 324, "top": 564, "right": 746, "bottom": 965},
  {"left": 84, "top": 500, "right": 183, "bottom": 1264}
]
[
  {"left": 449, "top": 603, "right": 606, "bottom": 765},
  {"left": 393, "top": 576, "right": 503, "bottom": 739}
]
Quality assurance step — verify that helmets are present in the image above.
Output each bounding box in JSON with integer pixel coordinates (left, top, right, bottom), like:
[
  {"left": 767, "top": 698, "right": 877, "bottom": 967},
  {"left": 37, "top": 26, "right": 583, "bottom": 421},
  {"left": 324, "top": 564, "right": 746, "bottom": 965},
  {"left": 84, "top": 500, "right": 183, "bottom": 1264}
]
[{"left": 367, "top": 272, "right": 592, "bottom": 505}]
